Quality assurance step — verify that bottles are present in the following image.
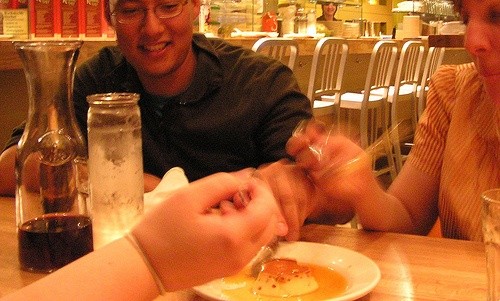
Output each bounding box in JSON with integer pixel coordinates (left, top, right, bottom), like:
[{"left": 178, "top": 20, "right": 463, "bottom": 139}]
[{"left": 12, "top": 41, "right": 94, "bottom": 274}]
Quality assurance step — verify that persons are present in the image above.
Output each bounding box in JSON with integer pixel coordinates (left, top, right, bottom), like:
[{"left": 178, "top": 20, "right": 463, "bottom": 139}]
[
  {"left": 285, "top": 0, "right": 500, "bottom": 244},
  {"left": 0, "top": 0, "right": 357, "bottom": 242},
  {"left": 318, "top": 3, "right": 341, "bottom": 21},
  {"left": 0, "top": 168, "right": 280, "bottom": 301}
]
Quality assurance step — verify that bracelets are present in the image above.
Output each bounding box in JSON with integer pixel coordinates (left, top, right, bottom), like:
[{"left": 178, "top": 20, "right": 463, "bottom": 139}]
[{"left": 125, "top": 232, "right": 165, "bottom": 296}]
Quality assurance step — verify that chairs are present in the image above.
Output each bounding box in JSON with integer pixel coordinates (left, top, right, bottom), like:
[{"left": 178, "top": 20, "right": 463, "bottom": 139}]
[{"left": 252, "top": 38, "right": 445, "bottom": 186}]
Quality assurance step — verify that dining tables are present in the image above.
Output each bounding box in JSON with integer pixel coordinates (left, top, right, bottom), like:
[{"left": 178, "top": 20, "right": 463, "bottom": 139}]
[{"left": 0, "top": 190, "right": 500, "bottom": 301}]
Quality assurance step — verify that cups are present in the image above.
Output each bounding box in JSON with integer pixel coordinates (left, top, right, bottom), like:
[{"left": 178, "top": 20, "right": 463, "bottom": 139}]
[
  {"left": 481, "top": 188, "right": 500, "bottom": 301},
  {"left": 87, "top": 92, "right": 144, "bottom": 250}
]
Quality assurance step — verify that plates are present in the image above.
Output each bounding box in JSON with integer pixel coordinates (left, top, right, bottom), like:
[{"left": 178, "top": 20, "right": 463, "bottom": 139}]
[{"left": 192, "top": 241, "right": 381, "bottom": 301}]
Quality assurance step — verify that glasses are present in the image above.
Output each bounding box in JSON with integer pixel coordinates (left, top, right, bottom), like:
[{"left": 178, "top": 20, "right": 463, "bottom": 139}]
[{"left": 110, "top": 0, "right": 191, "bottom": 23}]
